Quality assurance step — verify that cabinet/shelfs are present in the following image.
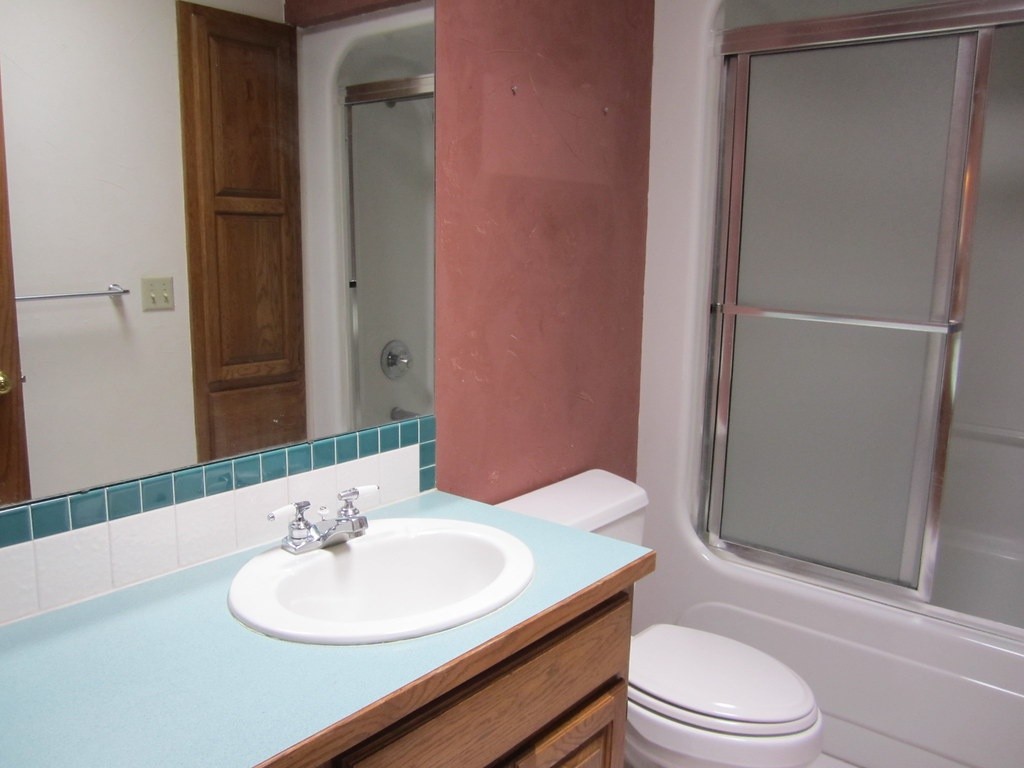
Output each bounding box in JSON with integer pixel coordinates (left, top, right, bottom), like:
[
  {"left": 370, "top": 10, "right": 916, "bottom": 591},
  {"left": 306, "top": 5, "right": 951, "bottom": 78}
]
[{"left": 328, "top": 583, "right": 635, "bottom": 768}]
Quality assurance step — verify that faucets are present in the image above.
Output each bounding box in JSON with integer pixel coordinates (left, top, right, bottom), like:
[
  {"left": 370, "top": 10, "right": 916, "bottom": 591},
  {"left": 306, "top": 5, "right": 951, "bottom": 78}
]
[{"left": 313, "top": 505, "right": 370, "bottom": 549}]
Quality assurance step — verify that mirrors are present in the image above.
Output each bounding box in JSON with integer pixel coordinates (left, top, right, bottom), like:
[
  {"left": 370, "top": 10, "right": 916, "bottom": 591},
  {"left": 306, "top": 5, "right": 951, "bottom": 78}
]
[{"left": 0, "top": 0, "right": 437, "bottom": 512}]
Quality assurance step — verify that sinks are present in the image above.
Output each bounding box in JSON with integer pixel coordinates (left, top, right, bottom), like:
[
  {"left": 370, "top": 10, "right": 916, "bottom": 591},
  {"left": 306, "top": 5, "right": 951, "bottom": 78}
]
[{"left": 225, "top": 516, "right": 536, "bottom": 646}]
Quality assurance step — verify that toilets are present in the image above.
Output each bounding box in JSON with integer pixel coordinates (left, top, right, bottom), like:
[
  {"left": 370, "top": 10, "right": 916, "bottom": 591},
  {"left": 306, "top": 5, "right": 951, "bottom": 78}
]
[{"left": 492, "top": 468, "right": 825, "bottom": 767}]
